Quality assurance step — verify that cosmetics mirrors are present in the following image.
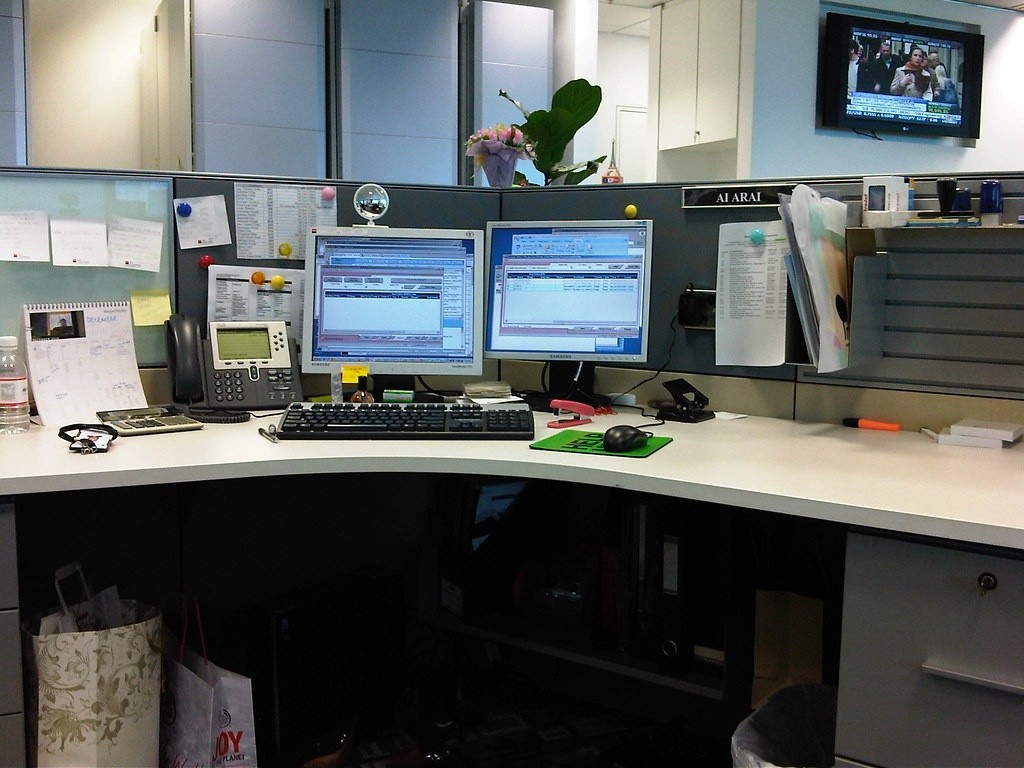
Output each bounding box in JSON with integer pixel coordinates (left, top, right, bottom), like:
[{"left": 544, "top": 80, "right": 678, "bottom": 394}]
[{"left": 352, "top": 183, "right": 391, "bottom": 229}]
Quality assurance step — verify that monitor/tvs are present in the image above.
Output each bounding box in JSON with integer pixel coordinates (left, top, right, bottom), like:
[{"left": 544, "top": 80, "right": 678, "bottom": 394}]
[
  {"left": 823, "top": 12, "right": 985, "bottom": 138},
  {"left": 301, "top": 225, "right": 485, "bottom": 403},
  {"left": 482, "top": 219, "right": 653, "bottom": 410}
]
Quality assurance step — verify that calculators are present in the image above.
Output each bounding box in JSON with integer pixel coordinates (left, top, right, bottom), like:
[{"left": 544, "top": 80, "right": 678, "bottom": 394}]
[{"left": 95, "top": 405, "right": 205, "bottom": 437}]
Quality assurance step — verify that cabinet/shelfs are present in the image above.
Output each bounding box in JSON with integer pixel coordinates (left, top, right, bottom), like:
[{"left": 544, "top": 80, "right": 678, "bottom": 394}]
[
  {"left": 833, "top": 525, "right": 1024, "bottom": 768},
  {"left": 657, "top": 0, "right": 743, "bottom": 152}
]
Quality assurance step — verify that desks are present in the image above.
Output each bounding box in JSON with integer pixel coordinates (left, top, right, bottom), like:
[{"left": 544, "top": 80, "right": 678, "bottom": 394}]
[
  {"left": 0, "top": 404, "right": 1024, "bottom": 552},
  {"left": 0, "top": 501, "right": 26, "bottom": 768}
]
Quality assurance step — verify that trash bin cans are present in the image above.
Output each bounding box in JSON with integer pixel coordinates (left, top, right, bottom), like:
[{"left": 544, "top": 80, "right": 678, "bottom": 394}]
[{"left": 730, "top": 680, "right": 840, "bottom": 768}]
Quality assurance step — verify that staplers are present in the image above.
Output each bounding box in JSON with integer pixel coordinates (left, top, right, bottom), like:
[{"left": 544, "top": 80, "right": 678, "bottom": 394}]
[{"left": 547, "top": 398, "right": 596, "bottom": 429}]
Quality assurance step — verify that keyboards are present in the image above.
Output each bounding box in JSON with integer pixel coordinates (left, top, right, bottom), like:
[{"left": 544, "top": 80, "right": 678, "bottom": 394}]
[{"left": 277, "top": 402, "right": 535, "bottom": 440}]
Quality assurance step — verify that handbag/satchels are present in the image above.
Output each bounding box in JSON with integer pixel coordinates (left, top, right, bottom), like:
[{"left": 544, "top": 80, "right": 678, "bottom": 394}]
[
  {"left": 20, "top": 560, "right": 162, "bottom": 768},
  {"left": 159, "top": 593, "right": 258, "bottom": 768}
]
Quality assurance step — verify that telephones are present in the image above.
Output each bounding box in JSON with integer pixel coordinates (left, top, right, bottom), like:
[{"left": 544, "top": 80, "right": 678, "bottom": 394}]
[{"left": 165, "top": 314, "right": 306, "bottom": 409}]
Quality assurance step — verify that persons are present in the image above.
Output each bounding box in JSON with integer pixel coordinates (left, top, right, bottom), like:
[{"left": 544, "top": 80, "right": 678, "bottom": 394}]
[
  {"left": 927, "top": 53, "right": 961, "bottom": 114},
  {"left": 889, "top": 44, "right": 942, "bottom": 102},
  {"left": 49, "top": 319, "right": 75, "bottom": 340},
  {"left": 866, "top": 42, "right": 904, "bottom": 95},
  {"left": 847, "top": 38, "right": 874, "bottom": 92}
]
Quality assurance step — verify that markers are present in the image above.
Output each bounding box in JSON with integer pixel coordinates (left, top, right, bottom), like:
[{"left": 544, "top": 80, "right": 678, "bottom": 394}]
[{"left": 842, "top": 417, "right": 901, "bottom": 431}]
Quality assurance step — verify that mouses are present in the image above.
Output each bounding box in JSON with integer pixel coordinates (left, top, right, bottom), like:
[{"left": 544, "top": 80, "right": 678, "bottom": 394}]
[{"left": 604, "top": 425, "right": 648, "bottom": 452}]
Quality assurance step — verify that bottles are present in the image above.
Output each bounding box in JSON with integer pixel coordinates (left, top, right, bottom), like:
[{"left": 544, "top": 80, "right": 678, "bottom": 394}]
[{"left": 0, "top": 335, "right": 30, "bottom": 435}]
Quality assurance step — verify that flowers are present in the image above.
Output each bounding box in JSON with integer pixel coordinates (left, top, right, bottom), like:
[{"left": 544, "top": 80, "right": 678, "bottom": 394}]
[{"left": 463, "top": 124, "right": 537, "bottom": 188}]
